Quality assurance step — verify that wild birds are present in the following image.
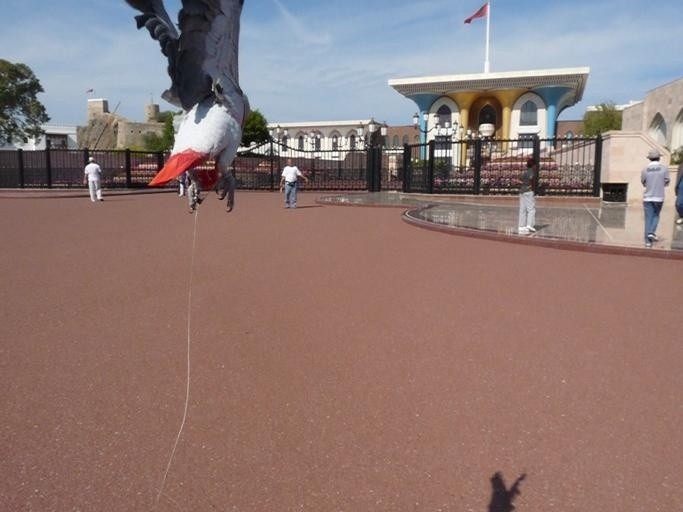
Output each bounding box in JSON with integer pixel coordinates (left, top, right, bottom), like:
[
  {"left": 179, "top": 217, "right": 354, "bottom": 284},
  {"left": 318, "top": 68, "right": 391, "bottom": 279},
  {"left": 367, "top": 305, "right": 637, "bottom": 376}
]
[{"left": 122, "top": 0, "right": 250, "bottom": 215}]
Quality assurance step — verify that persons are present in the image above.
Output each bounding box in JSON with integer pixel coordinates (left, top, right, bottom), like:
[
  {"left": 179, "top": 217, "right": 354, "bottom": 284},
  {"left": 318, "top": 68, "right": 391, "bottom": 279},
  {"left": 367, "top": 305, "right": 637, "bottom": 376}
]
[
  {"left": 83, "top": 156, "right": 104, "bottom": 203},
  {"left": 674, "top": 161, "right": 683, "bottom": 224},
  {"left": 516, "top": 156, "right": 539, "bottom": 233},
  {"left": 280, "top": 157, "right": 309, "bottom": 209},
  {"left": 639, "top": 149, "right": 671, "bottom": 247},
  {"left": 177, "top": 174, "right": 186, "bottom": 196}
]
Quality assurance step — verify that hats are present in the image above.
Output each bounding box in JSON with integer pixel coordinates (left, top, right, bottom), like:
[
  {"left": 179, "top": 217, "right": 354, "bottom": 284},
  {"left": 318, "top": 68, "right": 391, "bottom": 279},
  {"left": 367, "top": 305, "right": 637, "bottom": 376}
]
[
  {"left": 646, "top": 149, "right": 662, "bottom": 159},
  {"left": 89, "top": 157, "right": 94, "bottom": 161}
]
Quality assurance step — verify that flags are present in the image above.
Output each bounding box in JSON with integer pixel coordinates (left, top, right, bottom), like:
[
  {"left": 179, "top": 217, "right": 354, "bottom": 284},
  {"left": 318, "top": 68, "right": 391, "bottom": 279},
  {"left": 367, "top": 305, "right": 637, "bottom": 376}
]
[{"left": 464, "top": 1, "right": 491, "bottom": 24}]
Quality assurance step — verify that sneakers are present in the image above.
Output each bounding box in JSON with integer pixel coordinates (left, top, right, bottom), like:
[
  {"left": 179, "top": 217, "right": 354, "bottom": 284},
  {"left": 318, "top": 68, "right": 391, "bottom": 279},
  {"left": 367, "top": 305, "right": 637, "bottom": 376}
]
[
  {"left": 518, "top": 225, "right": 536, "bottom": 232},
  {"left": 646, "top": 233, "right": 658, "bottom": 247},
  {"left": 675, "top": 218, "right": 683, "bottom": 223}
]
